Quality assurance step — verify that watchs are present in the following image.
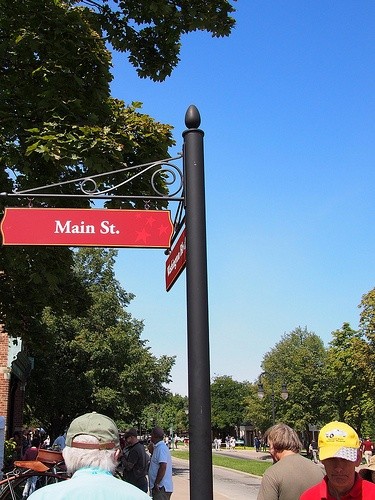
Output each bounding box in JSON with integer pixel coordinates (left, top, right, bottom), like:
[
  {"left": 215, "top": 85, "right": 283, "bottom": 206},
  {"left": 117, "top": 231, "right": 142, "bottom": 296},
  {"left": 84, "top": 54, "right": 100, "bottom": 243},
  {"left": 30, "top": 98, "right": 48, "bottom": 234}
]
[{"left": 154, "top": 483, "right": 159, "bottom": 486}]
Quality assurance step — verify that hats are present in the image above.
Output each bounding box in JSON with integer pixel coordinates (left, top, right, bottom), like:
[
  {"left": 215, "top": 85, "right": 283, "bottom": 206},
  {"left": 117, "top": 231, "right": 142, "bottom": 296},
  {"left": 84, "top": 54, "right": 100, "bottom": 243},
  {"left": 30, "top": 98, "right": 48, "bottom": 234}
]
[
  {"left": 122, "top": 428, "right": 138, "bottom": 436},
  {"left": 153, "top": 427, "right": 164, "bottom": 437},
  {"left": 318, "top": 420, "right": 359, "bottom": 461},
  {"left": 65, "top": 412, "right": 120, "bottom": 449}
]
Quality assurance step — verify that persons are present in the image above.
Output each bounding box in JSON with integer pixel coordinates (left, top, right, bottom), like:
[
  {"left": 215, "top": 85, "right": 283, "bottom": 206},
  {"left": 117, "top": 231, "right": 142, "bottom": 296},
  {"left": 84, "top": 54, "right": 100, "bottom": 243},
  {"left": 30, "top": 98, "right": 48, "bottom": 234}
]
[
  {"left": 22, "top": 438, "right": 42, "bottom": 500},
  {"left": 10, "top": 430, "right": 54, "bottom": 449},
  {"left": 359, "top": 436, "right": 374, "bottom": 467},
  {"left": 163, "top": 433, "right": 179, "bottom": 449},
  {"left": 309, "top": 439, "right": 318, "bottom": 460},
  {"left": 148, "top": 443, "right": 155, "bottom": 468},
  {"left": 26, "top": 411, "right": 153, "bottom": 500},
  {"left": 213, "top": 435, "right": 267, "bottom": 453},
  {"left": 148, "top": 427, "right": 173, "bottom": 500},
  {"left": 117, "top": 428, "right": 148, "bottom": 493},
  {"left": 257, "top": 423, "right": 326, "bottom": 500},
  {"left": 51, "top": 430, "right": 69, "bottom": 483},
  {"left": 300, "top": 421, "right": 375, "bottom": 500}
]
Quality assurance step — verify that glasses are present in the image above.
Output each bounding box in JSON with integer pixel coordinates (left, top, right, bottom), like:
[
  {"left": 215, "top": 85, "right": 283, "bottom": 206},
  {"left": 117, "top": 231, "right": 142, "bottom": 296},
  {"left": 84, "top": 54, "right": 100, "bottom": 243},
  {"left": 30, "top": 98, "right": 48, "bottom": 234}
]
[{"left": 126, "top": 434, "right": 131, "bottom": 437}]
